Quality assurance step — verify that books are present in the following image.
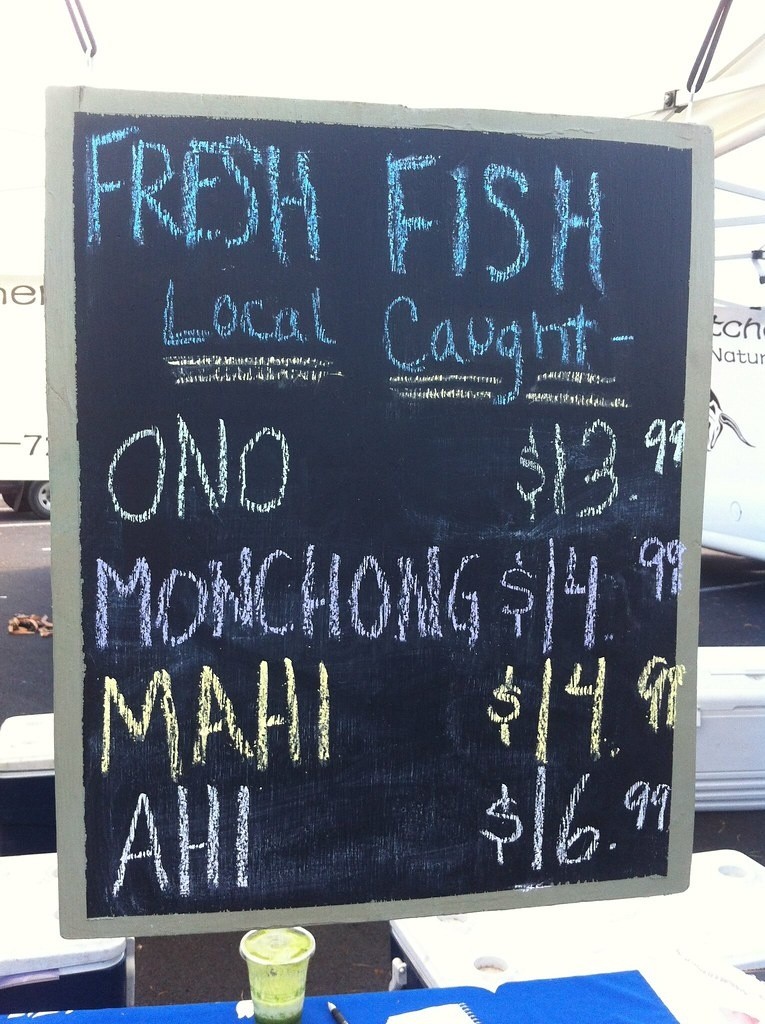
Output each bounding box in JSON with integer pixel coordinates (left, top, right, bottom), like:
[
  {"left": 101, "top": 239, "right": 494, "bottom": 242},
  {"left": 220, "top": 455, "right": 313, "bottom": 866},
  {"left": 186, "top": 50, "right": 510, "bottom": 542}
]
[{"left": 387, "top": 1002, "right": 480, "bottom": 1024}]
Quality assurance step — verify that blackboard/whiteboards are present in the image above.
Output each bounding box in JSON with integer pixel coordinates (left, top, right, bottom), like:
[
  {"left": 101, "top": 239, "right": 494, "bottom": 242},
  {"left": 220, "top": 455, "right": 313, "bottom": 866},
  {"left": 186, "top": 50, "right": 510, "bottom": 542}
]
[{"left": 41, "top": 80, "right": 715, "bottom": 939}]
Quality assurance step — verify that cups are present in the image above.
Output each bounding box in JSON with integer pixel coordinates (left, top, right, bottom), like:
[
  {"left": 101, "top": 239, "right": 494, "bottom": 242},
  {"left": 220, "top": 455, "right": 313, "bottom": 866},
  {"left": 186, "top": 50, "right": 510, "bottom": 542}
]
[{"left": 238, "top": 926, "right": 316, "bottom": 1024}]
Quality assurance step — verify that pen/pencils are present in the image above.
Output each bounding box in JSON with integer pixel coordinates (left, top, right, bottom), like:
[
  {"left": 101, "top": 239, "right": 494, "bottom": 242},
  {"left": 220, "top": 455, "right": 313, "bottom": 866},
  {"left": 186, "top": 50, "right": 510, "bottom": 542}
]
[{"left": 327, "top": 1001, "right": 348, "bottom": 1024}]
